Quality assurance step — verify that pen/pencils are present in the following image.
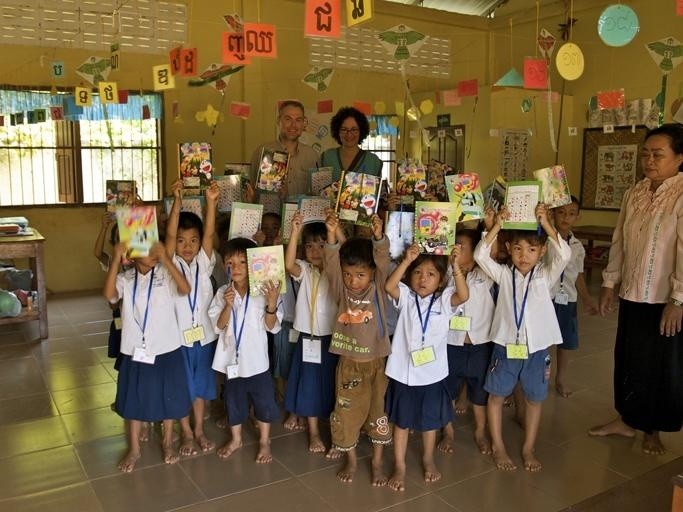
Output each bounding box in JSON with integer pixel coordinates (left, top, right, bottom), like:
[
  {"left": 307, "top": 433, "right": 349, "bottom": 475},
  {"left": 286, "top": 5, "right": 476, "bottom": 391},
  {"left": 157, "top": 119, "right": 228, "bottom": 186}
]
[
  {"left": 399, "top": 202, "right": 403, "bottom": 237},
  {"left": 537, "top": 200, "right": 543, "bottom": 236},
  {"left": 178, "top": 189, "right": 182, "bottom": 206},
  {"left": 316, "top": 161, "right": 318, "bottom": 168},
  {"left": 227, "top": 265, "right": 232, "bottom": 287}
]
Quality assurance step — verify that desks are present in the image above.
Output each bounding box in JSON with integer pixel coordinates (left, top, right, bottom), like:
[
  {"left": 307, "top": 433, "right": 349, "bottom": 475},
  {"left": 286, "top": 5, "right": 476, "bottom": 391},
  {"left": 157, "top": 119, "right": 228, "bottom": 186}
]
[
  {"left": 0, "top": 224, "right": 52, "bottom": 342},
  {"left": 568, "top": 223, "right": 616, "bottom": 289}
]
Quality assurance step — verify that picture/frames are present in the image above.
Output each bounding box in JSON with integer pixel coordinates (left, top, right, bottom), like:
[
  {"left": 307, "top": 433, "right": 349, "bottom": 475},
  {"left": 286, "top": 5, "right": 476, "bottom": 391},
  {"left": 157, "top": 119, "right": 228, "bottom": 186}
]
[{"left": 578, "top": 124, "right": 650, "bottom": 212}]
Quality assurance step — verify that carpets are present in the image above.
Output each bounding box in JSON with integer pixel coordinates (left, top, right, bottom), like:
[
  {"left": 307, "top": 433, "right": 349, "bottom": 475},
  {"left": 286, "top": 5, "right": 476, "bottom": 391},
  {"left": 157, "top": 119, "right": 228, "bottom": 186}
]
[{"left": 562, "top": 454, "right": 681, "bottom": 511}]
